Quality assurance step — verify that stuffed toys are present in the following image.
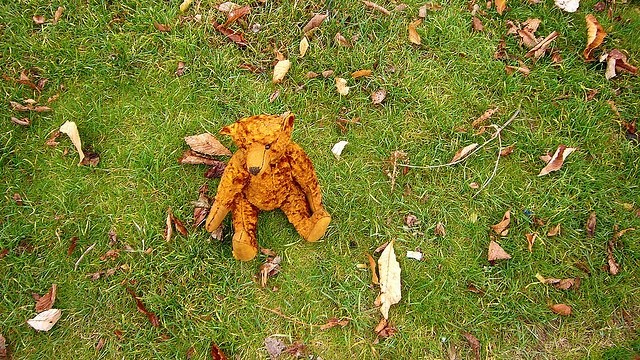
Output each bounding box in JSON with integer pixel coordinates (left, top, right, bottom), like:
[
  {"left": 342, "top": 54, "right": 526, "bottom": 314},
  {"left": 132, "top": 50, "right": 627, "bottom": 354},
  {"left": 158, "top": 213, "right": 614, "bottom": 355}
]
[{"left": 204, "top": 113, "right": 331, "bottom": 262}]
